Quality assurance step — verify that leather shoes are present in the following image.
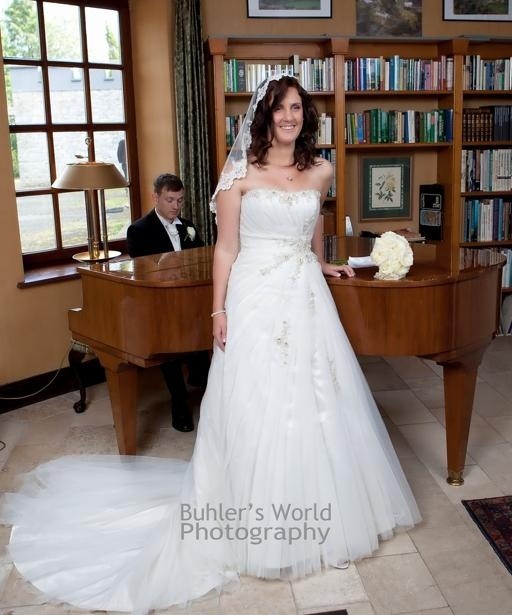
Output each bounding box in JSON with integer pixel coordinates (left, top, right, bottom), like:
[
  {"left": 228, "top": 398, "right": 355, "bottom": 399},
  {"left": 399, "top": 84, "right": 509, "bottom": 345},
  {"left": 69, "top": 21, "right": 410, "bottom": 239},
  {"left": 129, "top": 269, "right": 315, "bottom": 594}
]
[{"left": 172, "top": 401, "right": 194, "bottom": 432}]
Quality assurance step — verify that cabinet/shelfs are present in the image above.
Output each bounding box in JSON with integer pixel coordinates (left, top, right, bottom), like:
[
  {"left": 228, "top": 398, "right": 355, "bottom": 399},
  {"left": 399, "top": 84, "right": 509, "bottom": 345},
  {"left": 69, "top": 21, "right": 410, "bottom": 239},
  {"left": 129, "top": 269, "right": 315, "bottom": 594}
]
[
  {"left": 459, "top": 32, "right": 512, "bottom": 295},
  {"left": 210, "top": 38, "right": 339, "bottom": 235},
  {"left": 340, "top": 35, "right": 457, "bottom": 246}
]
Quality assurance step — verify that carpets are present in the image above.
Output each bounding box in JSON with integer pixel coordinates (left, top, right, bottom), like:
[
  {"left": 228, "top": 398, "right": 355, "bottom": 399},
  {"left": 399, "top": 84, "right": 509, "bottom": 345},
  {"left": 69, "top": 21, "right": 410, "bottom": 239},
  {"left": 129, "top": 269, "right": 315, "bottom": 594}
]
[{"left": 456, "top": 492, "right": 511, "bottom": 578}]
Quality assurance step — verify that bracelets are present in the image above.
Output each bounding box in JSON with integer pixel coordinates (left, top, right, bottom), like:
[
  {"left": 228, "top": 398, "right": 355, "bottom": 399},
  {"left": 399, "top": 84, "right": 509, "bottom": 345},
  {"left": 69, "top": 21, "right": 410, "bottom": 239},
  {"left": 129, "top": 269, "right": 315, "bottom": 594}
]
[{"left": 211, "top": 310, "right": 227, "bottom": 317}]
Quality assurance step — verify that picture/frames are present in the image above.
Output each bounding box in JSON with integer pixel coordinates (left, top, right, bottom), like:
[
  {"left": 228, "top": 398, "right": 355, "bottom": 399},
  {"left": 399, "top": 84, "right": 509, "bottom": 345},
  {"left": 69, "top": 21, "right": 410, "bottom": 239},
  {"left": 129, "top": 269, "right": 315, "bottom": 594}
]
[
  {"left": 361, "top": 155, "right": 412, "bottom": 221},
  {"left": 246, "top": 0, "right": 333, "bottom": 20},
  {"left": 442, "top": 0, "right": 512, "bottom": 23}
]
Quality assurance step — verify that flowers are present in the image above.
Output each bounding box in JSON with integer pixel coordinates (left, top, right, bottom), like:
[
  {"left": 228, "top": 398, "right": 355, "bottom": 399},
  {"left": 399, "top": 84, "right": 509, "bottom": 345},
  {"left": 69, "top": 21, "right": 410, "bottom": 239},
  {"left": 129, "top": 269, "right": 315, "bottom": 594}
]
[{"left": 326, "top": 230, "right": 415, "bottom": 281}]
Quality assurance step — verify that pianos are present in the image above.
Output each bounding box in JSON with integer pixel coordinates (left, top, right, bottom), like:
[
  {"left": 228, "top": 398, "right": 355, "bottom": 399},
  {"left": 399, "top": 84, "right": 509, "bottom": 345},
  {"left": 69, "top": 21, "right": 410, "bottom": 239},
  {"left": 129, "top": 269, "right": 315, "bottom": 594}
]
[{"left": 67, "top": 236, "right": 507, "bottom": 486}]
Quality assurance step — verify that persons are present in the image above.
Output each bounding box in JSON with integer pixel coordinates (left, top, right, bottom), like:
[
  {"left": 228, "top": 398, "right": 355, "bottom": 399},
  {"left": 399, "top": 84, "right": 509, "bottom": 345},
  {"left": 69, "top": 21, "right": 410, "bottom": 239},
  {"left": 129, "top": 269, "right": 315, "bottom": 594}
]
[
  {"left": 0, "top": 74, "right": 423, "bottom": 611},
  {"left": 127, "top": 173, "right": 212, "bottom": 432}
]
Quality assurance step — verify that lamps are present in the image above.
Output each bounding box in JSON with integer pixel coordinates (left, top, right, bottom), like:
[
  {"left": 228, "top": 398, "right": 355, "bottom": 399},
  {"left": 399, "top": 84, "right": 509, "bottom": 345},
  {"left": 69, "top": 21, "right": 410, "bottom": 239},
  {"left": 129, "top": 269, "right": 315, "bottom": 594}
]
[{"left": 50, "top": 159, "right": 136, "bottom": 264}]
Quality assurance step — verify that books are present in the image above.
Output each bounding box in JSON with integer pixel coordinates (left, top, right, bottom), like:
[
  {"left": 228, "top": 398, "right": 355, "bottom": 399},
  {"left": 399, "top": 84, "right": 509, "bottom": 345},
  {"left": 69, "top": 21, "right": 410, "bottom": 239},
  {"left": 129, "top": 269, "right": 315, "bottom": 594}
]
[{"left": 220, "top": 50, "right": 509, "bottom": 293}]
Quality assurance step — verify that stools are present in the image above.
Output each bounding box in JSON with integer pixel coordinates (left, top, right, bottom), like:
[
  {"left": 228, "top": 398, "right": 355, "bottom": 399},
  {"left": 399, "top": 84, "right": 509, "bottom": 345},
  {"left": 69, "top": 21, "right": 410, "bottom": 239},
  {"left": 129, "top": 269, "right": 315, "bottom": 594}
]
[{"left": 67, "top": 337, "right": 98, "bottom": 413}]
